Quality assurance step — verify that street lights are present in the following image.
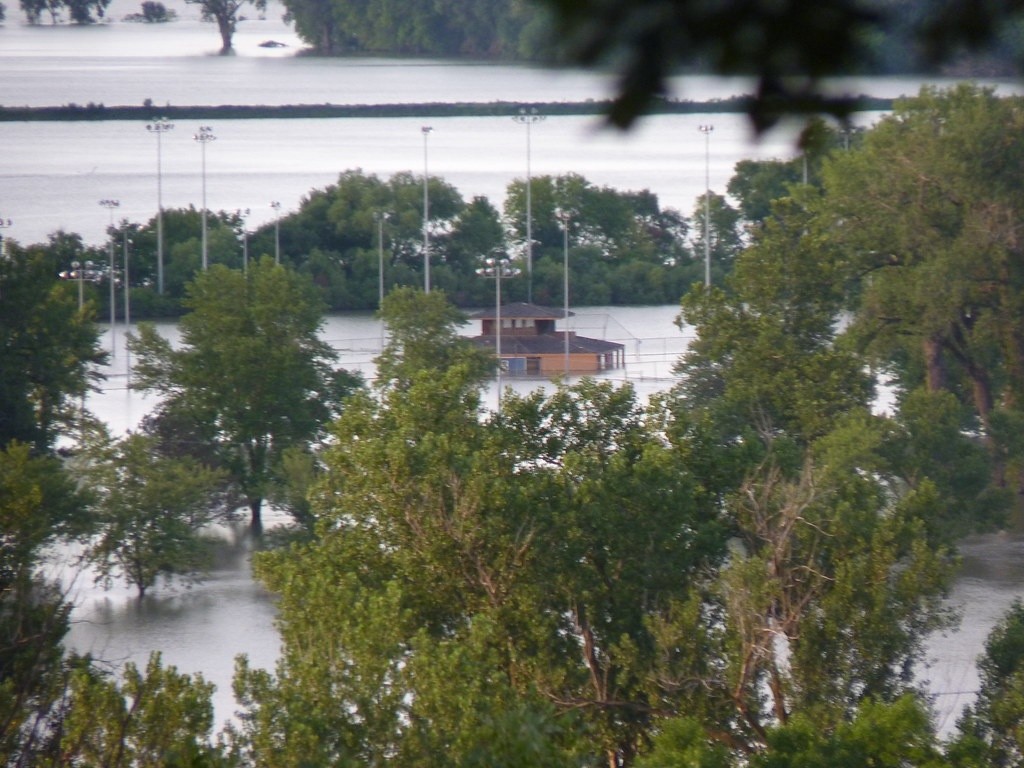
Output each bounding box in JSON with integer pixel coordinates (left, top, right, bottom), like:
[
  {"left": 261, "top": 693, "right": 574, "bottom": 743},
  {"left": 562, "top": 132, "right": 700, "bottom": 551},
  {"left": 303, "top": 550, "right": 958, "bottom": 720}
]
[{"left": 0, "top": 92, "right": 860, "bottom": 448}]
